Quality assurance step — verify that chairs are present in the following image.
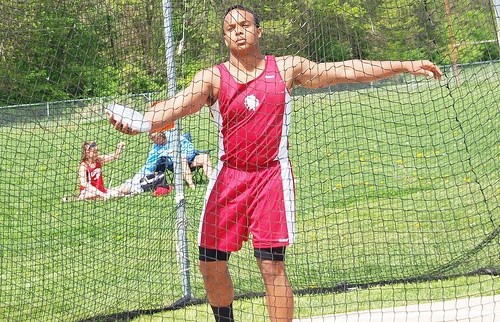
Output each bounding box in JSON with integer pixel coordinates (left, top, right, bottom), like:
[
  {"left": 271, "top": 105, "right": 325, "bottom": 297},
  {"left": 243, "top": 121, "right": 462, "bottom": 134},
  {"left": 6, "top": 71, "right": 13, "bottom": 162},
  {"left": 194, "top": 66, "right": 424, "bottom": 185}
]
[{"left": 156, "top": 131, "right": 215, "bottom": 186}]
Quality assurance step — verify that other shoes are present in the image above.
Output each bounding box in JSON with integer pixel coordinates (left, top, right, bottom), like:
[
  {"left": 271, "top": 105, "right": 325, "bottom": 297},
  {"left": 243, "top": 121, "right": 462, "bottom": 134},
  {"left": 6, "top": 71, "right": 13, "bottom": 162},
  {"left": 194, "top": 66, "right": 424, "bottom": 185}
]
[{"left": 63, "top": 196, "right": 77, "bottom": 202}]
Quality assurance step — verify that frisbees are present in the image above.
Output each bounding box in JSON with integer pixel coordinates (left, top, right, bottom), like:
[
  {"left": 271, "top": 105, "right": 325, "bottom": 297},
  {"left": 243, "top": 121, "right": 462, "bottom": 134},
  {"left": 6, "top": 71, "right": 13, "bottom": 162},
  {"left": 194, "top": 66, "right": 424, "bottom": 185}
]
[{"left": 107, "top": 104, "right": 152, "bottom": 133}]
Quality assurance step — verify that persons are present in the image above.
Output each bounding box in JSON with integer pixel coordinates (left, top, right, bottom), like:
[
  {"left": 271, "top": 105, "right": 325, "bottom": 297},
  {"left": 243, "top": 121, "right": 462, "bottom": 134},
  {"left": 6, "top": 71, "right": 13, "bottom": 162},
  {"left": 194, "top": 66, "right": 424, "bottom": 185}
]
[
  {"left": 105, "top": 5, "right": 443, "bottom": 322},
  {"left": 77, "top": 131, "right": 214, "bottom": 200}
]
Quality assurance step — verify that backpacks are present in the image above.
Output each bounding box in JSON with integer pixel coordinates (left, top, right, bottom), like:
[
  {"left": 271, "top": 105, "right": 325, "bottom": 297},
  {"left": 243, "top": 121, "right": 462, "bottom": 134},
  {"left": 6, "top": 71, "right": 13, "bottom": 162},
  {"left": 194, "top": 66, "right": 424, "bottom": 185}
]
[{"left": 140, "top": 170, "right": 167, "bottom": 192}]
